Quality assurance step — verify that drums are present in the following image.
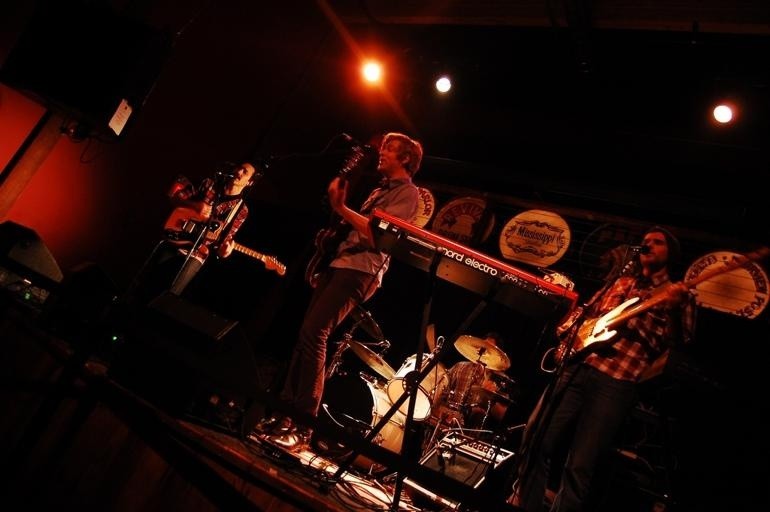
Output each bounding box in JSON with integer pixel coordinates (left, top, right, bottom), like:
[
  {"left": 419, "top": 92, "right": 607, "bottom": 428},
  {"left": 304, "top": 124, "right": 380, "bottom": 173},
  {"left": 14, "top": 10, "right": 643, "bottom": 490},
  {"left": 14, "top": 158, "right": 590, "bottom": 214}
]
[
  {"left": 388, "top": 353, "right": 447, "bottom": 421},
  {"left": 317, "top": 371, "right": 406, "bottom": 472}
]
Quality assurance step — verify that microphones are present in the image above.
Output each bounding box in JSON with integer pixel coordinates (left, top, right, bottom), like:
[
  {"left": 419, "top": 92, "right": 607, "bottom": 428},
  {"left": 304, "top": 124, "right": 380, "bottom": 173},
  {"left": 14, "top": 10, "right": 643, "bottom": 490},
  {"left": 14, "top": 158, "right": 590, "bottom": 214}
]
[
  {"left": 340, "top": 133, "right": 376, "bottom": 155},
  {"left": 216, "top": 170, "right": 235, "bottom": 179},
  {"left": 627, "top": 246, "right": 649, "bottom": 255}
]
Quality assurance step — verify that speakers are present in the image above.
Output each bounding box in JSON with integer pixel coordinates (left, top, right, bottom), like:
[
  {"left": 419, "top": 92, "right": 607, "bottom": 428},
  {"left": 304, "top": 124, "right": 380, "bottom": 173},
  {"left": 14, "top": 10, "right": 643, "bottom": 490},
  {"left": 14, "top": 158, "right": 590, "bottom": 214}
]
[{"left": 114, "top": 287, "right": 252, "bottom": 417}]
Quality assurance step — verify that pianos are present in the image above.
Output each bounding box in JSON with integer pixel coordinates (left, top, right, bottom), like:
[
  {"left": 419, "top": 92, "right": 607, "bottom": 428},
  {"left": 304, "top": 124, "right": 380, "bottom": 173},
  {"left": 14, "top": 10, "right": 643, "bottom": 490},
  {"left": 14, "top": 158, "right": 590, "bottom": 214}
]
[{"left": 367, "top": 204, "right": 579, "bottom": 330}]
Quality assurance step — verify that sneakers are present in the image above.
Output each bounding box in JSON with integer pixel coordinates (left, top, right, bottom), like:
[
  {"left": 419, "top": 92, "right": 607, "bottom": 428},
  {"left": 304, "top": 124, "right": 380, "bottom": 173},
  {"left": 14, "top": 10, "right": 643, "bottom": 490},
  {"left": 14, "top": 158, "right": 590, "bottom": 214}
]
[
  {"left": 245, "top": 429, "right": 272, "bottom": 445},
  {"left": 267, "top": 427, "right": 314, "bottom": 449}
]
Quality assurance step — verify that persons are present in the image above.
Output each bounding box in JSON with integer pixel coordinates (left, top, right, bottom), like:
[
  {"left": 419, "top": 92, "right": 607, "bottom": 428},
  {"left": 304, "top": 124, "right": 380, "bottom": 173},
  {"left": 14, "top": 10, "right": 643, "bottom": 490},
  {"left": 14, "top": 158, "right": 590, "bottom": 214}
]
[
  {"left": 96, "top": 153, "right": 266, "bottom": 380},
  {"left": 256, "top": 131, "right": 423, "bottom": 448},
  {"left": 519, "top": 227, "right": 697, "bottom": 512}
]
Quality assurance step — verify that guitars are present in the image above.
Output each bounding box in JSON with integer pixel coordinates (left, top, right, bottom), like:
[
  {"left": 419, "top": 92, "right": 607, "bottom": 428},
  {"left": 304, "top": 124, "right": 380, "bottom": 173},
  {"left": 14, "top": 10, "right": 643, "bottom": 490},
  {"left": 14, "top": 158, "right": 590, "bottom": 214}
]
[
  {"left": 162, "top": 207, "right": 288, "bottom": 275},
  {"left": 554, "top": 247, "right": 770, "bottom": 362},
  {"left": 303, "top": 144, "right": 374, "bottom": 287}
]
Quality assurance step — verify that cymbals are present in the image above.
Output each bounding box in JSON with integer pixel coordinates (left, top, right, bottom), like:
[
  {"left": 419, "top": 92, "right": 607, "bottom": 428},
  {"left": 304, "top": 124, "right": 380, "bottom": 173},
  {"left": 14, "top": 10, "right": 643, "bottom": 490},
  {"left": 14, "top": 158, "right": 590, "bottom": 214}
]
[
  {"left": 350, "top": 306, "right": 384, "bottom": 342},
  {"left": 454, "top": 336, "right": 510, "bottom": 371},
  {"left": 491, "top": 371, "right": 515, "bottom": 384},
  {"left": 471, "top": 385, "right": 515, "bottom": 405},
  {"left": 346, "top": 341, "right": 396, "bottom": 380}
]
